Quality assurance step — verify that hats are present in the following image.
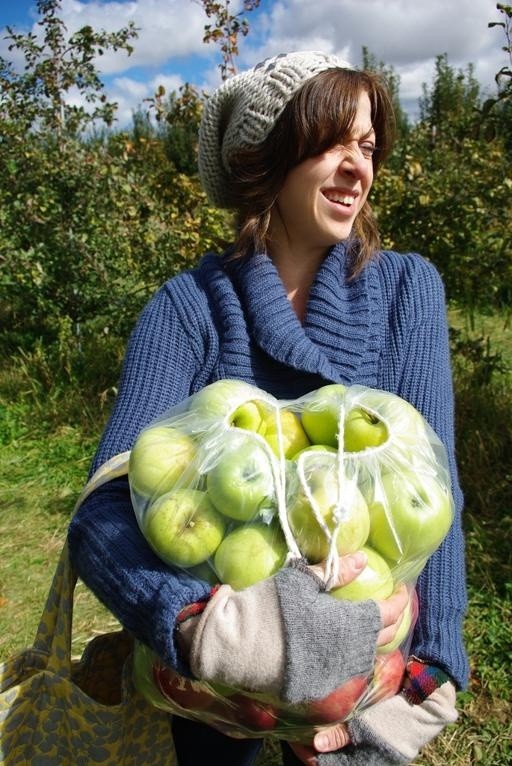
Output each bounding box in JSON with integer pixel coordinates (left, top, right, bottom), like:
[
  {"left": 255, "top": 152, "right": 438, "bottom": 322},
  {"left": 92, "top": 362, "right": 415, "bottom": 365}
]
[{"left": 200, "top": 52, "right": 360, "bottom": 210}]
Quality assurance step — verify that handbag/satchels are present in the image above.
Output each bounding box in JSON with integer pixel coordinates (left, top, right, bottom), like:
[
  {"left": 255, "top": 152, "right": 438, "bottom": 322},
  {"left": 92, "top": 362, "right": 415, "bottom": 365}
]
[{"left": 0, "top": 631, "right": 179, "bottom": 766}]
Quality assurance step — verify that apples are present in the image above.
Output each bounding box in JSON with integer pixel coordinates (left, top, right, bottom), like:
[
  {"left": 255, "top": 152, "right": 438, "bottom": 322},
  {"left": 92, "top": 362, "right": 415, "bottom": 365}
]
[{"left": 128, "top": 379, "right": 457, "bottom": 731}]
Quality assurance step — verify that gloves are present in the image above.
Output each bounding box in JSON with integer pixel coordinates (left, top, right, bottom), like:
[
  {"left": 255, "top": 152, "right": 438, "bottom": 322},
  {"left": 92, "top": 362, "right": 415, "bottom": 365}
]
[
  {"left": 319, "top": 680, "right": 459, "bottom": 765},
  {"left": 189, "top": 561, "right": 381, "bottom": 703}
]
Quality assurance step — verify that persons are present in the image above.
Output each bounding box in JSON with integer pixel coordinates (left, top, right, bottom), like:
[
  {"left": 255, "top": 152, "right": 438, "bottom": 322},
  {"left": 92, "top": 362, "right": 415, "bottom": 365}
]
[{"left": 66, "top": 50, "right": 470, "bottom": 766}]
